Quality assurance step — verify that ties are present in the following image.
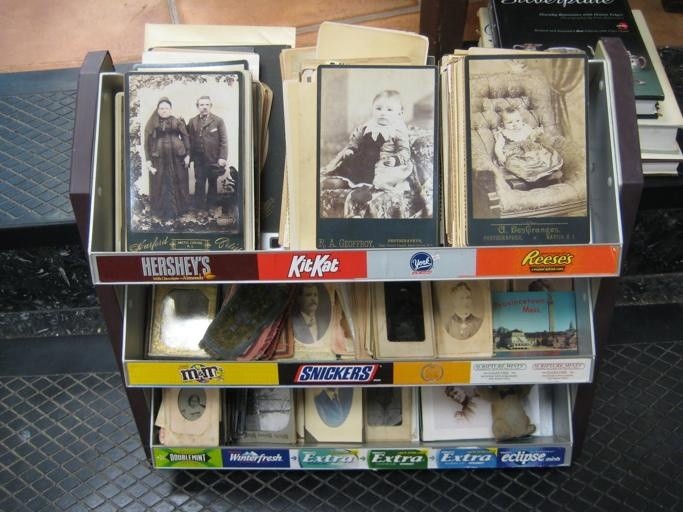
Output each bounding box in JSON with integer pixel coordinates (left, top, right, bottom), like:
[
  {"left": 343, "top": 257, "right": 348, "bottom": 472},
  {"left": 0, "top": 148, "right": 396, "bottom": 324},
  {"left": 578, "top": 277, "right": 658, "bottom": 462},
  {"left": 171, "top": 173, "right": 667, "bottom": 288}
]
[{"left": 332, "top": 393, "right": 339, "bottom": 410}]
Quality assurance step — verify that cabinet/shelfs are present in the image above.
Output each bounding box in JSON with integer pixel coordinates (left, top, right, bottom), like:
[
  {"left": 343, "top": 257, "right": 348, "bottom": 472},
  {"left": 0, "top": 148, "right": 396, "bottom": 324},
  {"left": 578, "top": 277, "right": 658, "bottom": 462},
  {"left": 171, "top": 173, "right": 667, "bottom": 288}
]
[{"left": 65, "top": 41, "right": 639, "bottom": 474}]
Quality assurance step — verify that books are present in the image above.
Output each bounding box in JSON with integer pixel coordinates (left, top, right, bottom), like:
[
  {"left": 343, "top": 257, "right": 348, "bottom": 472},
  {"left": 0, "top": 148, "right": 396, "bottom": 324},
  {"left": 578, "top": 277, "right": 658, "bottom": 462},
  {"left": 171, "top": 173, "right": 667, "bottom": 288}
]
[
  {"left": 124, "top": 20, "right": 589, "bottom": 252},
  {"left": 477, "top": 0, "right": 683, "bottom": 176},
  {"left": 145, "top": 281, "right": 579, "bottom": 360},
  {"left": 155, "top": 383, "right": 555, "bottom": 448}
]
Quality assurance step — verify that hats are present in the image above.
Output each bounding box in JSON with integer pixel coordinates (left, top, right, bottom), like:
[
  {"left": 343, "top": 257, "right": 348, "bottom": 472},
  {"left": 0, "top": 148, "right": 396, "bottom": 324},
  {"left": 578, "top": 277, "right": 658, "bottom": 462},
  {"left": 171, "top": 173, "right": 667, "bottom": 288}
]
[
  {"left": 160, "top": 97, "right": 168, "bottom": 101},
  {"left": 200, "top": 96, "right": 209, "bottom": 99}
]
